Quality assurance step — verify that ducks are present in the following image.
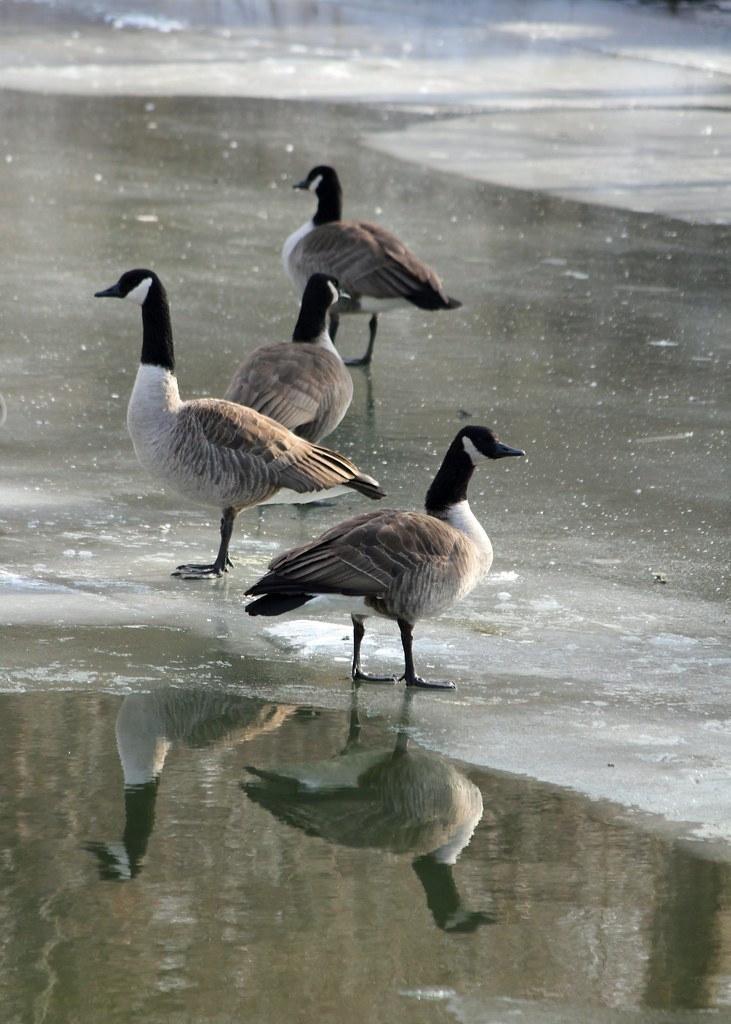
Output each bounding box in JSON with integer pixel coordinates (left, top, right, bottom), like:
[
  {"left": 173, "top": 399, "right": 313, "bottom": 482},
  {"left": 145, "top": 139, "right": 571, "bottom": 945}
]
[{"left": 219, "top": 271, "right": 352, "bottom": 507}]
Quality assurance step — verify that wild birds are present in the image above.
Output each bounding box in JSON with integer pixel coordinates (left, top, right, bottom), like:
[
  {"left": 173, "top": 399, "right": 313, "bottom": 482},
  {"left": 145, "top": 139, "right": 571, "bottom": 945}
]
[
  {"left": 281, "top": 163, "right": 462, "bottom": 367},
  {"left": 237, "top": 681, "right": 502, "bottom": 933},
  {"left": 76, "top": 693, "right": 314, "bottom": 883},
  {"left": 243, "top": 424, "right": 525, "bottom": 689},
  {"left": 93, "top": 269, "right": 387, "bottom": 580}
]
